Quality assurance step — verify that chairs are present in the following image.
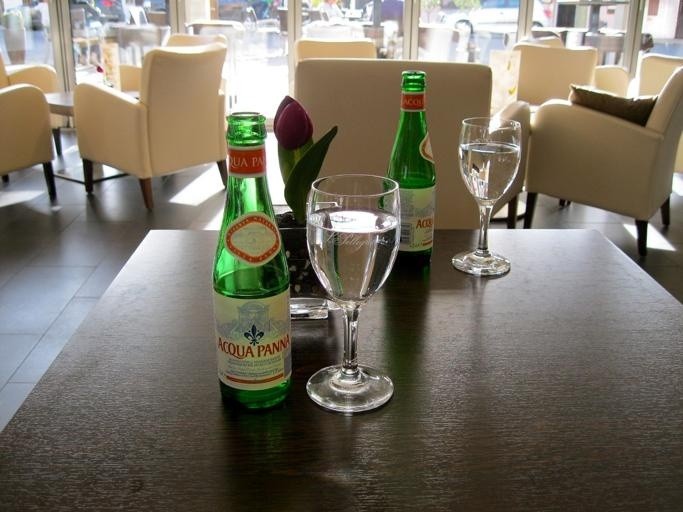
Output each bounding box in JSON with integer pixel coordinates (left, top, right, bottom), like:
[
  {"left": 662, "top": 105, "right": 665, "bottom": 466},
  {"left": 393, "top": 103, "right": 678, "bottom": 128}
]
[
  {"left": 296, "top": 40, "right": 376, "bottom": 60},
  {"left": 592, "top": 52, "right": 683, "bottom": 175},
  {"left": 0, "top": 49, "right": 62, "bottom": 202},
  {"left": 118, "top": 33, "right": 226, "bottom": 92},
  {"left": 511, "top": 42, "right": 630, "bottom": 112},
  {"left": 525, "top": 66, "right": 683, "bottom": 258},
  {"left": 294, "top": 58, "right": 531, "bottom": 228},
  {"left": 73, "top": 41, "right": 228, "bottom": 210}
]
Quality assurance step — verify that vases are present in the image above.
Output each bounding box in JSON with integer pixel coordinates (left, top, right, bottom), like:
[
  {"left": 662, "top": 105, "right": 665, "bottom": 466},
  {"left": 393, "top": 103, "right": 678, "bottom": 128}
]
[{"left": 274, "top": 228, "right": 338, "bottom": 320}]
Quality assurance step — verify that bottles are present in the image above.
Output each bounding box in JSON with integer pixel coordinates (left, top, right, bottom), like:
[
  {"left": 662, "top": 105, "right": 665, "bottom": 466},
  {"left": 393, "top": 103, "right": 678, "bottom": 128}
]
[
  {"left": 384, "top": 70, "right": 436, "bottom": 264},
  {"left": 213, "top": 113, "right": 290, "bottom": 408}
]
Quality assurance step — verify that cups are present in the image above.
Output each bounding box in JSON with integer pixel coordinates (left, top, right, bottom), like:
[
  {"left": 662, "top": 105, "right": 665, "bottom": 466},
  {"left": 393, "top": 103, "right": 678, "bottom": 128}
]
[
  {"left": 451, "top": 117, "right": 523, "bottom": 278},
  {"left": 305, "top": 172, "right": 401, "bottom": 414}
]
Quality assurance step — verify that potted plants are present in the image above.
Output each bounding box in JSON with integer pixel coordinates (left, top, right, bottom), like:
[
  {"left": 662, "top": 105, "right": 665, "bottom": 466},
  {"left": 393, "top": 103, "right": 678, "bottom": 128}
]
[{"left": 3, "top": 9, "right": 26, "bottom": 65}]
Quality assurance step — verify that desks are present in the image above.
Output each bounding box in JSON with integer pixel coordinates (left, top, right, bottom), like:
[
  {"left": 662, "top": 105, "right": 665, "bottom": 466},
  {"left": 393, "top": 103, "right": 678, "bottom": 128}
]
[{"left": 0, "top": 227, "right": 681, "bottom": 512}]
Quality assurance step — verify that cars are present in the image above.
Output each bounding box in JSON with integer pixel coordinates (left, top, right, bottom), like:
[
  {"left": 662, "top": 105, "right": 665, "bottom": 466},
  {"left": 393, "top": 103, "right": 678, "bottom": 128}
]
[{"left": 439, "top": 0, "right": 553, "bottom": 37}]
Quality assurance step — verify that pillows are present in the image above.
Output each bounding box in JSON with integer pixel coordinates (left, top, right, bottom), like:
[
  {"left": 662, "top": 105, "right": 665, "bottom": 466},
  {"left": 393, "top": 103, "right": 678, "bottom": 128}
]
[{"left": 567, "top": 82, "right": 657, "bottom": 127}]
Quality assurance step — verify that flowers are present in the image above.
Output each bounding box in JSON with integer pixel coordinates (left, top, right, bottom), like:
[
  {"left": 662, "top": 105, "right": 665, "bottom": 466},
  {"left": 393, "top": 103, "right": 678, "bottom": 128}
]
[{"left": 275, "top": 95, "right": 339, "bottom": 226}]
